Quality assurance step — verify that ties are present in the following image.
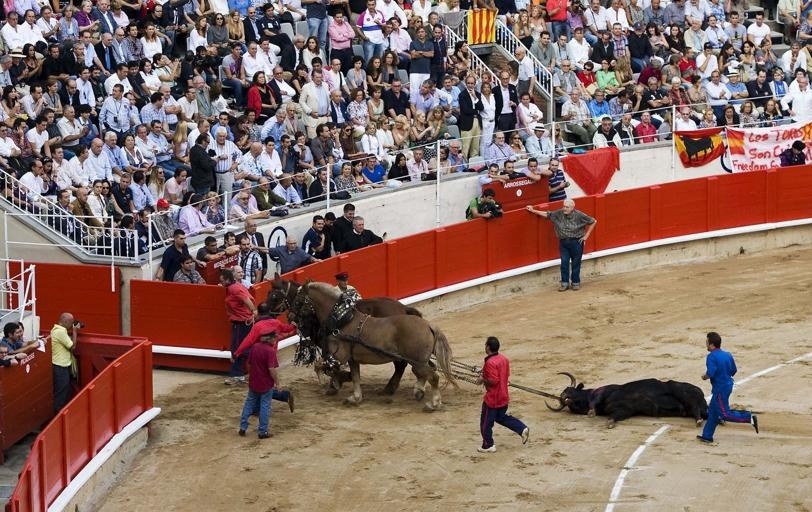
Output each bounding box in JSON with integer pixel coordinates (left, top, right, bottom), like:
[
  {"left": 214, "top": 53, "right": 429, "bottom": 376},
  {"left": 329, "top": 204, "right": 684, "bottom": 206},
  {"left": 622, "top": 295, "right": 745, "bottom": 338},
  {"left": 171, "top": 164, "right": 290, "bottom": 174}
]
[
  {"left": 251, "top": 236, "right": 257, "bottom": 247},
  {"left": 105, "top": 47, "right": 111, "bottom": 69}
]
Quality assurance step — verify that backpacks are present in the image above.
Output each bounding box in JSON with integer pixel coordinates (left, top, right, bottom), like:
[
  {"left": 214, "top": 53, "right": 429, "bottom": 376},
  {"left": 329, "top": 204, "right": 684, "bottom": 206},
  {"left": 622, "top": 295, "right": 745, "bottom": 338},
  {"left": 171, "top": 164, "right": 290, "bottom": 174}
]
[{"left": 465, "top": 205, "right": 470, "bottom": 218}]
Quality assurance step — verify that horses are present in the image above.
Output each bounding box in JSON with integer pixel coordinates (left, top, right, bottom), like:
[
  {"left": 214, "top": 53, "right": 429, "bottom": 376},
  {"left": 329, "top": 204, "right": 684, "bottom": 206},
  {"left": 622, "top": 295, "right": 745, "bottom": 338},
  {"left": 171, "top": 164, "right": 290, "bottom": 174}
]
[
  {"left": 287, "top": 279, "right": 462, "bottom": 412},
  {"left": 267, "top": 272, "right": 422, "bottom": 395}
]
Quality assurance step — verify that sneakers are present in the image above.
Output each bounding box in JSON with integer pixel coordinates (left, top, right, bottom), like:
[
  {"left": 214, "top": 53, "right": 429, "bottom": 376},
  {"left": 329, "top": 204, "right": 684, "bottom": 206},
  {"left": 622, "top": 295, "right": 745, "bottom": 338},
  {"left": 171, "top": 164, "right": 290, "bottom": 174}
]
[
  {"left": 238, "top": 428, "right": 245, "bottom": 436},
  {"left": 521, "top": 426, "right": 529, "bottom": 444},
  {"left": 751, "top": 414, "right": 759, "bottom": 434},
  {"left": 289, "top": 391, "right": 295, "bottom": 413},
  {"left": 558, "top": 284, "right": 569, "bottom": 291},
  {"left": 571, "top": 282, "right": 580, "bottom": 290},
  {"left": 225, "top": 376, "right": 239, "bottom": 385},
  {"left": 696, "top": 435, "right": 713, "bottom": 443},
  {"left": 477, "top": 445, "right": 496, "bottom": 453},
  {"left": 258, "top": 432, "right": 273, "bottom": 439}
]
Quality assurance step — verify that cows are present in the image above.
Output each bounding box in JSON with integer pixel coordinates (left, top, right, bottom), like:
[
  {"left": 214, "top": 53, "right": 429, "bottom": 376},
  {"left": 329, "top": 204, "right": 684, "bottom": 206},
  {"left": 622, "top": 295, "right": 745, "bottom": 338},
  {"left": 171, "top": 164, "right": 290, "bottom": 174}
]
[
  {"left": 679, "top": 134, "right": 714, "bottom": 163},
  {"left": 544, "top": 372, "right": 710, "bottom": 429}
]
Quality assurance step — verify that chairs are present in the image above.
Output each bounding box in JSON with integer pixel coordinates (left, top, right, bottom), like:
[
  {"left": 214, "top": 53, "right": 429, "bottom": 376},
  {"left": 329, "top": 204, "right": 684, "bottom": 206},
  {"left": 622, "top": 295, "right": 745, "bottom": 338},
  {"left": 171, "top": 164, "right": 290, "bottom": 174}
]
[
  {"left": 544, "top": 20, "right": 642, "bottom": 131},
  {"left": 775, "top": 3, "right": 786, "bottom": 25},
  {"left": 183, "top": 11, "right": 410, "bottom": 93},
  {"left": 446, "top": 124, "right": 461, "bottom": 138},
  {"left": 796, "top": 28, "right": 802, "bottom": 41}
]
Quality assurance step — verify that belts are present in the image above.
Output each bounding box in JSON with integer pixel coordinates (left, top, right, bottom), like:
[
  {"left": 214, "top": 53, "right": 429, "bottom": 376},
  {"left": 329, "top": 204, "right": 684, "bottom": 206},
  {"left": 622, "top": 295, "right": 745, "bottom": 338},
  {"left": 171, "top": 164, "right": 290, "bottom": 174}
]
[{"left": 216, "top": 169, "right": 230, "bottom": 174}]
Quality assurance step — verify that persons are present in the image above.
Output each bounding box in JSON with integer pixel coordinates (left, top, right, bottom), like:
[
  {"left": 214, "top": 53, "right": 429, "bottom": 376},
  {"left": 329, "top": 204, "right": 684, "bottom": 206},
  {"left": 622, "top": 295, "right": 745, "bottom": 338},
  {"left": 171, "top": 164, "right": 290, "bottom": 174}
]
[
  {"left": 18, "top": 321, "right": 50, "bottom": 346},
  {"left": 695, "top": 332, "right": 759, "bottom": 444},
  {"left": 50, "top": 312, "right": 82, "bottom": 412},
  {"left": 474, "top": 335, "right": 530, "bottom": 453},
  {"left": 236, "top": 326, "right": 281, "bottom": 438},
  {"left": 1, "top": 320, "right": 44, "bottom": 356},
  {"left": 331, "top": 271, "right": 361, "bottom": 303},
  {"left": 0, "top": 0, "right": 812, "bottom": 291},
  {"left": 218, "top": 269, "right": 257, "bottom": 385},
  {"left": 231, "top": 301, "right": 296, "bottom": 385},
  {"left": 1, "top": 345, "right": 27, "bottom": 367}
]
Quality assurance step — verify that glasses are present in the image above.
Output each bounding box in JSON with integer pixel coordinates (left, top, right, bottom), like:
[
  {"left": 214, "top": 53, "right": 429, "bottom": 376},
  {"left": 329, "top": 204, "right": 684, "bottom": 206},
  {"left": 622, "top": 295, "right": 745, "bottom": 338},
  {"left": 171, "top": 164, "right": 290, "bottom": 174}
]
[
  {"left": 344, "top": 128, "right": 353, "bottom": 132},
  {"left": 416, "top": 19, "right": 423, "bottom": 24},
  {"left": 102, "top": 186, "right": 110, "bottom": 189},
  {"left": 583, "top": 66, "right": 592, "bottom": 72},
  {"left": 513, "top": 137, "right": 521, "bottom": 140},
  {"left": 240, "top": 197, "right": 250, "bottom": 201},
  {"left": 249, "top": 11, "right": 256, "bottom": 15},
  {"left": 216, "top": 17, "right": 224, "bottom": 21},
  {"left": 158, "top": 171, "right": 165, "bottom": 175}
]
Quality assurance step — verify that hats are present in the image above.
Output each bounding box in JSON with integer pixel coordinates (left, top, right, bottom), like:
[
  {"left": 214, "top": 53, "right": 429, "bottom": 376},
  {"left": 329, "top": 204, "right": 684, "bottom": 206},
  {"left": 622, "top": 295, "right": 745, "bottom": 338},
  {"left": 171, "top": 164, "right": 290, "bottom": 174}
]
[
  {"left": 772, "top": 66, "right": 785, "bottom": 79},
  {"left": 157, "top": 198, "right": 170, "bottom": 208},
  {"left": 634, "top": 22, "right": 644, "bottom": 30},
  {"left": 600, "top": 114, "right": 612, "bottom": 121},
  {"left": 259, "top": 327, "right": 279, "bottom": 338},
  {"left": 792, "top": 140, "right": 807, "bottom": 150},
  {"left": 8, "top": 47, "right": 27, "bottom": 58},
  {"left": 704, "top": 42, "right": 713, "bottom": 50},
  {"left": 335, "top": 271, "right": 350, "bottom": 281},
  {"left": 532, "top": 122, "right": 547, "bottom": 132},
  {"left": 725, "top": 68, "right": 741, "bottom": 78}
]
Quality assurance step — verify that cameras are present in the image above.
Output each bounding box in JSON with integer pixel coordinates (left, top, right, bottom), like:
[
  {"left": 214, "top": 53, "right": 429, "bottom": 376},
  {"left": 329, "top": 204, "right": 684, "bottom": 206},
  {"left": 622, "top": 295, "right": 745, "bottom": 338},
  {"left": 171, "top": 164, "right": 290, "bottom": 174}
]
[{"left": 74, "top": 319, "right": 85, "bottom": 328}]
